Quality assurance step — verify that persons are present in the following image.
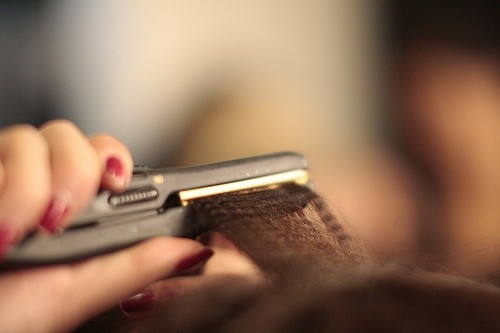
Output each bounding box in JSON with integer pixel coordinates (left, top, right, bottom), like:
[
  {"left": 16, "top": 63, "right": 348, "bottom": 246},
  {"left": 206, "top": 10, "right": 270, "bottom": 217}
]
[{"left": 1, "top": 119, "right": 500, "bottom": 333}]
